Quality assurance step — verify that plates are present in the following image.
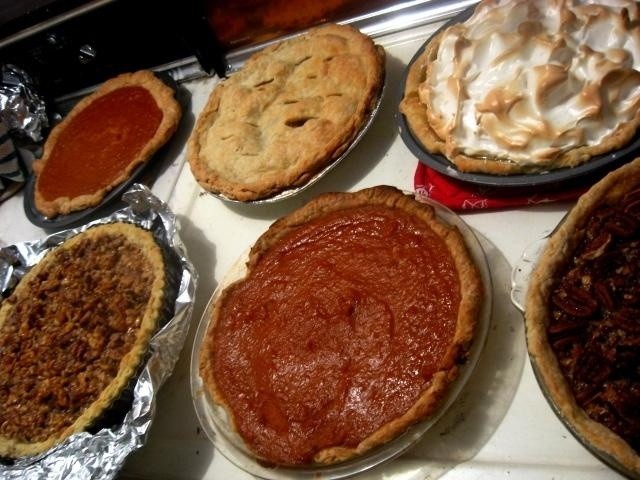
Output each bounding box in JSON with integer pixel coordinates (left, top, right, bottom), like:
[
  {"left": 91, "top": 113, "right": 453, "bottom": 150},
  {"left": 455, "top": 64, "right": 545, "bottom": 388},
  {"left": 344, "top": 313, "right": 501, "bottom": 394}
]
[{"left": 21, "top": 73, "right": 181, "bottom": 231}]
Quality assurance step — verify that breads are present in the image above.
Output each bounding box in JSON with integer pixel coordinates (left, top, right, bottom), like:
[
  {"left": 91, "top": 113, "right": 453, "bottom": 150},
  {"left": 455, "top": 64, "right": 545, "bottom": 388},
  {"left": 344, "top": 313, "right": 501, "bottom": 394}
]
[
  {"left": 0, "top": 221, "right": 181, "bottom": 466},
  {"left": 32, "top": 70, "right": 183, "bottom": 219},
  {"left": 525, "top": 157, "right": 640, "bottom": 478}
]
